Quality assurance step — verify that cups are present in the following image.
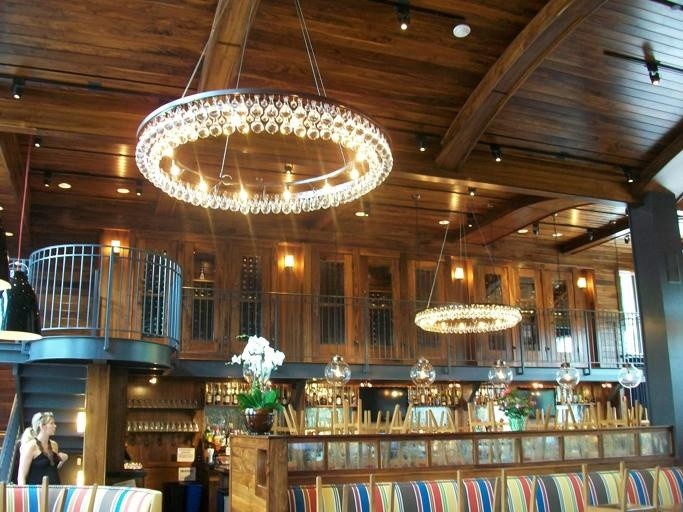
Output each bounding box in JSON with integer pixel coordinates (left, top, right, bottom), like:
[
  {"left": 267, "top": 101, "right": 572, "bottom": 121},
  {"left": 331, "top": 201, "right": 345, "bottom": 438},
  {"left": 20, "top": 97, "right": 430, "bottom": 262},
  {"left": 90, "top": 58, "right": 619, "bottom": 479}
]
[
  {"left": 126, "top": 421, "right": 199, "bottom": 431},
  {"left": 126, "top": 399, "right": 199, "bottom": 409}
]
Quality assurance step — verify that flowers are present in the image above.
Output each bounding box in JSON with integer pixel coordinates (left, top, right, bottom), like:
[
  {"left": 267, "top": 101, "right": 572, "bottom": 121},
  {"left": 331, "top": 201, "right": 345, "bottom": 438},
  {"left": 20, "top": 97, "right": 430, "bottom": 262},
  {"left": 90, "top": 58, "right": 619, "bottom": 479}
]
[
  {"left": 490, "top": 379, "right": 537, "bottom": 421},
  {"left": 222, "top": 328, "right": 288, "bottom": 411}
]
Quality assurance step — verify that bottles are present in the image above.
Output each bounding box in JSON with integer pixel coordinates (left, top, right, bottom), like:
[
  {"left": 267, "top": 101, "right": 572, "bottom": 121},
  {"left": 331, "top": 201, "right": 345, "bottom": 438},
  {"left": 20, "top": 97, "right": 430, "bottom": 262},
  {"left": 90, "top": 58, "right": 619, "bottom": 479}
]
[
  {"left": 411, "top": 388, "right": 461, "bottom": 406},
  {"left": 205, "top": 420, "right": 249, "bottom": 463},
  {"left": 206, "top": 384, "right": 288, "bottom": 405},
  {"left": 304, "top": 389, "right": 358, "bottom": 405}
]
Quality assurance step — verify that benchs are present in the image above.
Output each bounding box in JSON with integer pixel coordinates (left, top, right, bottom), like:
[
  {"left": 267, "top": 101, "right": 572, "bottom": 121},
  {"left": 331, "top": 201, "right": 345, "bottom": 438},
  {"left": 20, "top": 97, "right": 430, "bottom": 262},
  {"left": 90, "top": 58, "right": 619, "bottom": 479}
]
[{"left": 1, "top": 479, "right": 164, "bottom": 511}]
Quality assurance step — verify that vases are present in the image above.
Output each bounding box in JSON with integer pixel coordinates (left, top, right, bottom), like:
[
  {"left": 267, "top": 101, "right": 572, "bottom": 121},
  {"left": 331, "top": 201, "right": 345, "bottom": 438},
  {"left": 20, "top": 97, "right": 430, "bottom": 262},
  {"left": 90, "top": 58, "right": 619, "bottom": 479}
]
[{"left": 242, "top": 408, "right": 274, "bottom": 437}]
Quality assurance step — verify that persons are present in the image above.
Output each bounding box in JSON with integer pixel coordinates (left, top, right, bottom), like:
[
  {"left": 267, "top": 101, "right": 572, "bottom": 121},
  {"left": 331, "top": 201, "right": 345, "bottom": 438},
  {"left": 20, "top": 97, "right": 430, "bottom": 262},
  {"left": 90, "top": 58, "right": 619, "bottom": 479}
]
[
  {"left": 17, "top": 411, "right": 68, "bottom": 485},
  {"left": 194, "top": 423, "right": 220, "bottom": 512}
]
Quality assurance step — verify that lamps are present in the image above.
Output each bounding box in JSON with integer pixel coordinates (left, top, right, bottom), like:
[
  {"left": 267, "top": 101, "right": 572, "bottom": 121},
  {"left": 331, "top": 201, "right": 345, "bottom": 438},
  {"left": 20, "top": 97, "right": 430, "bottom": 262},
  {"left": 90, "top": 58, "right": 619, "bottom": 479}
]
[
  {"left": 0, "top": 70, "right": 143, "bottom": 202},
  {"left": 110, "top": 239, "right": 120, "bottom": 257},
  {"left": 283, "top": 254, "right": 293, "bottom": 271},
  {"left": 416, "top": 135, "right": 504, "bottom": 229},
  {"left": 412, "top": 187, "right": 522, "bottom": 335},
  {"left": 131, "top": 2, "right": 392, "bottom": 221},
  {"left": 454, "top": 267, "right": 463, "bottom": 280}
]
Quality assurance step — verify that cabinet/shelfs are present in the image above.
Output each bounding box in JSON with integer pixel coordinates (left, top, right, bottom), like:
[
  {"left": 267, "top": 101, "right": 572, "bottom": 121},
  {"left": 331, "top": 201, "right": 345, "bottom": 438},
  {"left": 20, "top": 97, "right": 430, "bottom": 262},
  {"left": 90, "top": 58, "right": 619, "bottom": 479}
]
[
  {"left": 302, "top": 381, "right": 361, "bottom": 407},
  {"left": 191, "top": 278, "right": 215, "bottom": 303},
  {"left": 367, "top": 288, "right": 391, "bottom": 312},
  {"left": 224, "top": 424, "right": 677, "bottom": 511},
  {"left": 408, "top": 383, "right": 464, "bottom": 408},
  {"left": 124, "top": 405, "right": 198, "bottom": 437},
  {"left": 202, "top": 381, "right": 290, "bottom": 407}
]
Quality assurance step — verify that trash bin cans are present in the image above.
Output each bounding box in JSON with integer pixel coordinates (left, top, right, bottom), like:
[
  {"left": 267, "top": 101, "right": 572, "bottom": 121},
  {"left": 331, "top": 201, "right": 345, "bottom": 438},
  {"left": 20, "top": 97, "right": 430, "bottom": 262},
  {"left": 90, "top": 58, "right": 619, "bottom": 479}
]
[
  {"left": 183, "top": 480, "right": 204, "bottom": 512},
  {"left": 216, "top": 488, "right": 228, "bottom": 512}
]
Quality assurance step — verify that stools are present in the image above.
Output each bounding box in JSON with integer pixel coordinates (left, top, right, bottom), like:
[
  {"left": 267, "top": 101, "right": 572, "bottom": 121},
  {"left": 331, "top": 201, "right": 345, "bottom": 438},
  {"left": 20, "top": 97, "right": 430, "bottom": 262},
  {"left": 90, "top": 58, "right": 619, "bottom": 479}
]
[{"left": 271, "top": 399, "right": 683, "bottom": 511}]
